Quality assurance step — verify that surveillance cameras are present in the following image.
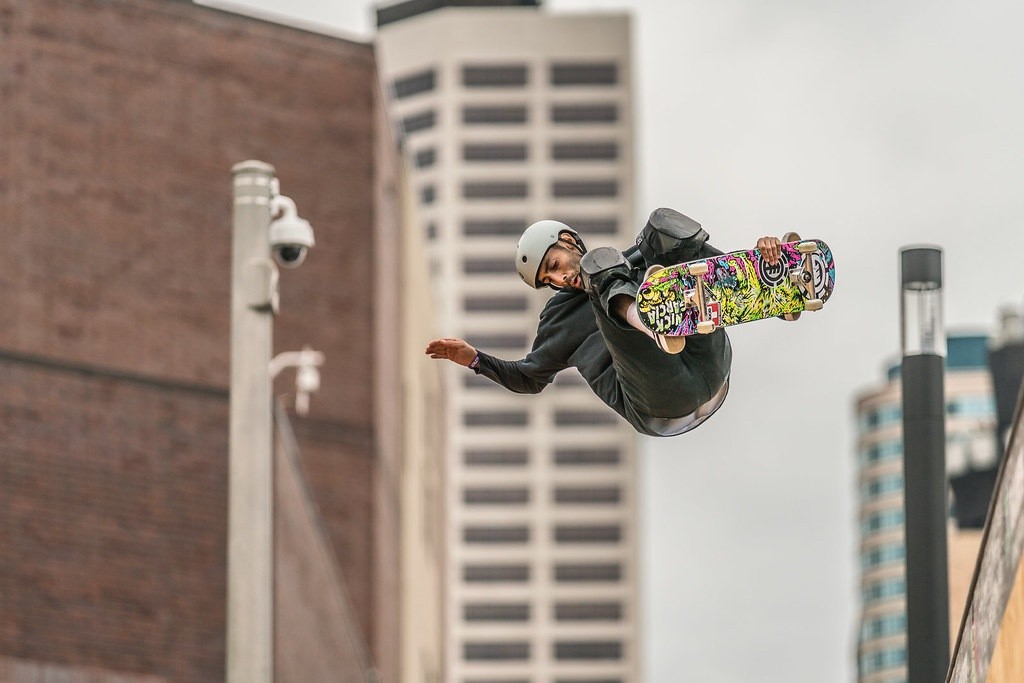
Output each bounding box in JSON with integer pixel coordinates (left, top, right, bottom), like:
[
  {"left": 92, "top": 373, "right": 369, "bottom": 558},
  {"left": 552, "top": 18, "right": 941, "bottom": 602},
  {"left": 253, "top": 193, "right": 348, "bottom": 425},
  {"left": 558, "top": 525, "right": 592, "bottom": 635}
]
[{"left": 268, "top": 217, "right": 315, "bottom": 269}]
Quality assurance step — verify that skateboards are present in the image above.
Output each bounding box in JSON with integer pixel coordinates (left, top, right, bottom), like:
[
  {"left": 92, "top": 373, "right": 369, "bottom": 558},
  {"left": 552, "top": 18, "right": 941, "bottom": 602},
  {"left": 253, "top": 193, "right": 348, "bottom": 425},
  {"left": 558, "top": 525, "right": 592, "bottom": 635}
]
[{"left": 634, "top": 235, "right": 837, "bottom": 337}]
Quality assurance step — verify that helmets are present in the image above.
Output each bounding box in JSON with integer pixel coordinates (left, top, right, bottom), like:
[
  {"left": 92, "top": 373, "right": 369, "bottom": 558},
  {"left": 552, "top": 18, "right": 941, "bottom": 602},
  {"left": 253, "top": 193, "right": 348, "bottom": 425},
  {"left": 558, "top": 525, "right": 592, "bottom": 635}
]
[{"left": 514, "top": 220, "right": 578, "bottom": 289}]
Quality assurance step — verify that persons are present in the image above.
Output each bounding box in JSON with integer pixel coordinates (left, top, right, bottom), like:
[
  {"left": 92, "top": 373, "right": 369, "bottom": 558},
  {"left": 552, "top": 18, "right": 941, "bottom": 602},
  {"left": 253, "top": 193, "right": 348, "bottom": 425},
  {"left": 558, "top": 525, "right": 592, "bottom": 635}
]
[{"left": 425, "top": 208, "right": 803, "bottom": 438}]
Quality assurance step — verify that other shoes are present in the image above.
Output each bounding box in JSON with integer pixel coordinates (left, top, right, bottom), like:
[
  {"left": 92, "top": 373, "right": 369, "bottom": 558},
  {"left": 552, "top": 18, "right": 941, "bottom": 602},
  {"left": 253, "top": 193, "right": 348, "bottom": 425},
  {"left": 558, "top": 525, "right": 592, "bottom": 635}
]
[
  {"left": 777, "top": 231, "right": 802, "bottom": 321},
  {"left": 643, "top": 264, "right": 686, "bottom": 355}
]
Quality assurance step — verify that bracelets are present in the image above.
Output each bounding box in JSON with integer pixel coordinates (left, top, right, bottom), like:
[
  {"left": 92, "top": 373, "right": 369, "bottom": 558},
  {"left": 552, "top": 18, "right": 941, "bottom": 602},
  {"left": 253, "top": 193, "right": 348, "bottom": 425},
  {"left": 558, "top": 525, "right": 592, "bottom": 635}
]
[{"left": 468, "top": 357, "right": 480, "bottom": 369}]
